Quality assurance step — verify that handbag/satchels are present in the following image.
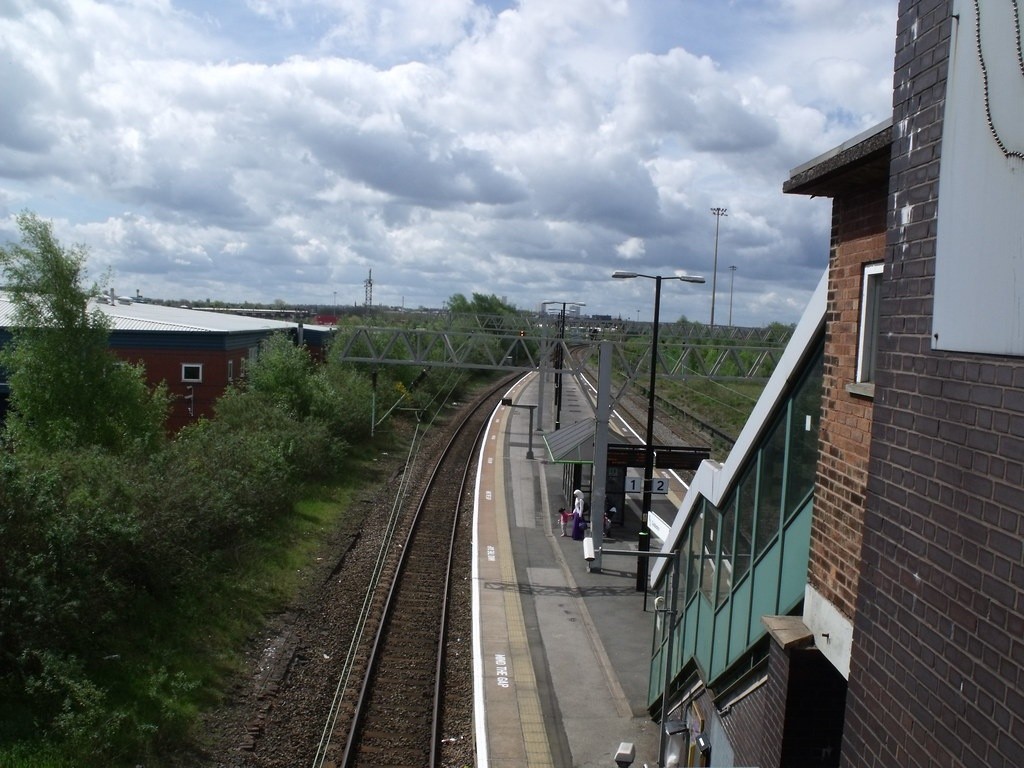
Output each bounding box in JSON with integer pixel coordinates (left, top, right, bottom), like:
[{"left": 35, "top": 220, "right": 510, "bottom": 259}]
[{"left": 578, "top": 517, "right": 587, "bottom": 531}]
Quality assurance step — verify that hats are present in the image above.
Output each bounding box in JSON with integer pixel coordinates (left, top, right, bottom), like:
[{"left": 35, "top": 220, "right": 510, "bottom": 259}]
[{"left": 574, "top": 490, "right": 584, "bottom": 499}]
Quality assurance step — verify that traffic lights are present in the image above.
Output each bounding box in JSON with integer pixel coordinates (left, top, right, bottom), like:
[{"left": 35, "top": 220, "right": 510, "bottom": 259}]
[{"left": 520, "top": 331, "right": 524, "bottom": 336}]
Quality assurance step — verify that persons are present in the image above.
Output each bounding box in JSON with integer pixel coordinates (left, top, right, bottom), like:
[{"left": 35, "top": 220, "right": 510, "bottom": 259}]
[
  {"left": 603, "top": 494, "right": 612, "bottom": 538},
  {"left": 572, "top": 490, "right": 584, "bottom": 540},
  {"left": 559, "top": 508, "right": 568, "bottom": 537}
]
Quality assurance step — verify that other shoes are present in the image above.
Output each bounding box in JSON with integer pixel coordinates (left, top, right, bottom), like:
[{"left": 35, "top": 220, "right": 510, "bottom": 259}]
[
  {"left": 561, "top": 533, "right": 567, "bottom": 536},
  {"left": 574, "top": 538, "right": 580, "bottom": 540}
]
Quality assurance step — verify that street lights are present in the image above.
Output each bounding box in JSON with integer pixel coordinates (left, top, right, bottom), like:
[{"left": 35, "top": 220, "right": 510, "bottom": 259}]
[
  {"left": 543, "top": 300, "right": 587, "bottom": 431},
  {"left": 549, "top": 308, "right": 576, "bottom": 405},
  {"left": 611, "top": 270, "right": 707, "bottom": 592}
]
